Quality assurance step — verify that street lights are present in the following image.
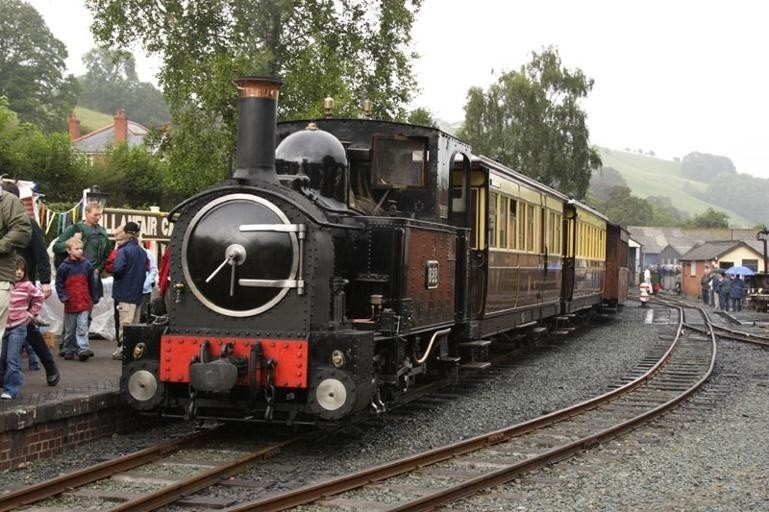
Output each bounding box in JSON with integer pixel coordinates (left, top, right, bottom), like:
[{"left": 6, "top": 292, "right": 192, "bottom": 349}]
[{"left": 84, "top": 184, "right": 109, "bottom": 208}]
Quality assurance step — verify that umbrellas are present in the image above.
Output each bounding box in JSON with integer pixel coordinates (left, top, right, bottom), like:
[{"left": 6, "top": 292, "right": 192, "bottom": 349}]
[{"left": 724, "top": 265, "right": 756, "bottom": 276}]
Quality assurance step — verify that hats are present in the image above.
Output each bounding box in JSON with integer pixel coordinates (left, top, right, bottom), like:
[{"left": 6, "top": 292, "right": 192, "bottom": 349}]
[{"left": 124, "top": 222, "right": 140, "bottom": 233}]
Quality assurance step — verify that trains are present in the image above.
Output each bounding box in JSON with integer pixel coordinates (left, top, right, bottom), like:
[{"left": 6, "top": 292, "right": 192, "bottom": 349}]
[{"left": 110, "top": 73, "right": 632, "bottom": 438}]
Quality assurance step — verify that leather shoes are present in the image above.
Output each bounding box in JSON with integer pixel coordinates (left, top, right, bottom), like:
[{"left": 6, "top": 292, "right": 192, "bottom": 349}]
[{"left": 48, "top": 370, "right": 59, "bottom": 386}]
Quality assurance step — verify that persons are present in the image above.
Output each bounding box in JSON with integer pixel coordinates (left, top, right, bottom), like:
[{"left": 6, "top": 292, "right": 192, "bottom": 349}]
[
  {"left": 701, "top": 268, "right": 745, "bottom": 313},
  {"left": 0, "top": 179, "right": 160, "bottom": 397}
]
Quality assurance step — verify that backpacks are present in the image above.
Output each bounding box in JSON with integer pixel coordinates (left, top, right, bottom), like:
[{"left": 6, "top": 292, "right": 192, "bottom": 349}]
[{"left": 54, "top": 224, "right": 80, "bottom": 268}]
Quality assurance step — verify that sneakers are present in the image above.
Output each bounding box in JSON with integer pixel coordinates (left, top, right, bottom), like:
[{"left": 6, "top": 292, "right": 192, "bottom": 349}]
[
  {"left": 112, "top": 345, "right": 124, "bottom": 359},
  {"left": 60, "top": 346, "right": 94, "bottom": 361},
  {"left": 0, "top": 393, "right": 12, "bottom": 399}
]
